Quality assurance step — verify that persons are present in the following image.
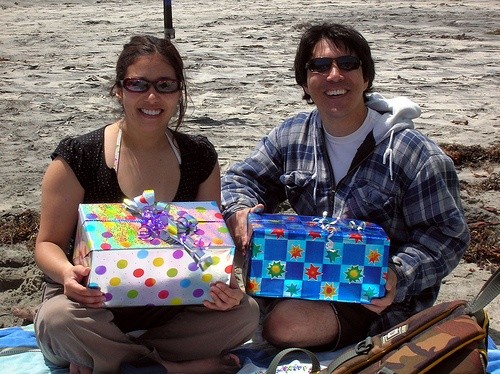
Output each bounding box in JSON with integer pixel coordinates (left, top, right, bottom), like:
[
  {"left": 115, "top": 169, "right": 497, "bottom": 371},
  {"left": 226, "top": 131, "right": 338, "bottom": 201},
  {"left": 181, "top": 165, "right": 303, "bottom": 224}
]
[
  {"left": 221, "top": 23, "right": 472, "bottom": 354},
  {"left": 32, "top": 33, "right": 261, "bottom": 374}
]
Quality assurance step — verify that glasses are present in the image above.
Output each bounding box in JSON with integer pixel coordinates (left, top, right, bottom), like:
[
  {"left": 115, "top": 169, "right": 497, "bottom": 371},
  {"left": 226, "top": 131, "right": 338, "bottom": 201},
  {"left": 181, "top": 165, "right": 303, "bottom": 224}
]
[
  {"left": 305, "top": 54, "right": 364, "bottom": 72},
  {"left": 118, "top": 77, "right": 184, "bottom": 94}
]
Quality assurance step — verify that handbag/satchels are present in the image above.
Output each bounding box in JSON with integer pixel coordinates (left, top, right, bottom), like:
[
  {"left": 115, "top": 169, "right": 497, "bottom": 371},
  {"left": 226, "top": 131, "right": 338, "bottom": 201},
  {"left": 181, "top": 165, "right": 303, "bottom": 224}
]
[{"left": 268, "top": 297, "right": 490, "bottom": 374}]
